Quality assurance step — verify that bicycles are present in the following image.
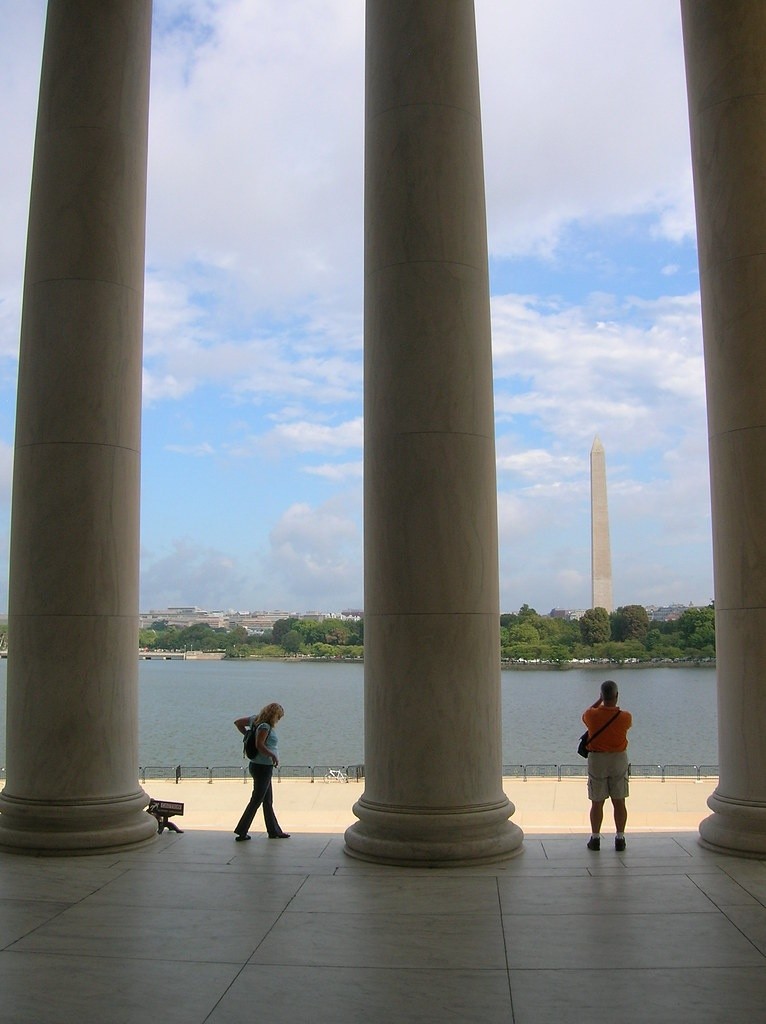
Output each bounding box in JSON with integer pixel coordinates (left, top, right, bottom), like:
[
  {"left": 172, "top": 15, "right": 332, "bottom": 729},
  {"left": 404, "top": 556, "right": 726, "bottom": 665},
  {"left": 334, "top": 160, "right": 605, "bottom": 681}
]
[{"left": 323, "top": 766, "right": 349, "bottom": 783}]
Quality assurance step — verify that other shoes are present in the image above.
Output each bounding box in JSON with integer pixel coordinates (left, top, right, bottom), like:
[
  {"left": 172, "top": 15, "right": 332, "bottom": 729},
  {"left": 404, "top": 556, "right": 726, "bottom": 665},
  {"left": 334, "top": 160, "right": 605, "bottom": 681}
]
[
  {"left": 235, "top": 834, "right": 251, "bottom": 841},
  {"left": 615, "top": 836, "right": 626, "bottom": 851},
  {"left": 268, "top": 832, "right": 291, "bottom": 838},
  {"left": 587, "top": 836, "right": 600, "bottom": 851}
]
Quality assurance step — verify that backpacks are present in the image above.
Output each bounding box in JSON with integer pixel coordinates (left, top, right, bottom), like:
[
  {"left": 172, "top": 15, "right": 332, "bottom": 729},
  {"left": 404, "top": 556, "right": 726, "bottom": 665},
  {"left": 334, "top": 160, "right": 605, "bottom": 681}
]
[{"left": 242, "top": 719, "right": 271, "bottom": 759}]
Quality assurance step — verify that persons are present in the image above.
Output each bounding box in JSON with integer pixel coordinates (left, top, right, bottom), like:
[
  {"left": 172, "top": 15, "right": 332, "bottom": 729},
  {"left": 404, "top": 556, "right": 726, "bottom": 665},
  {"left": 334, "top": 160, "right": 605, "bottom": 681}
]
[
  {"left": 234, "top": 703, "right": 291, "bottom": 841},
  {"left": 581, "top": 681, "right": 632, "bottom": 852}
]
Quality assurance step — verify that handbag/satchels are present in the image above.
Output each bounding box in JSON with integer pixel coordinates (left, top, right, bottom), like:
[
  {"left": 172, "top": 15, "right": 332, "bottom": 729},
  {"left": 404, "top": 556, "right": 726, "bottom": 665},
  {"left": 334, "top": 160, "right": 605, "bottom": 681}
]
[{"left": 577, "top": 710, "right": 624, "bottom": 758}]
